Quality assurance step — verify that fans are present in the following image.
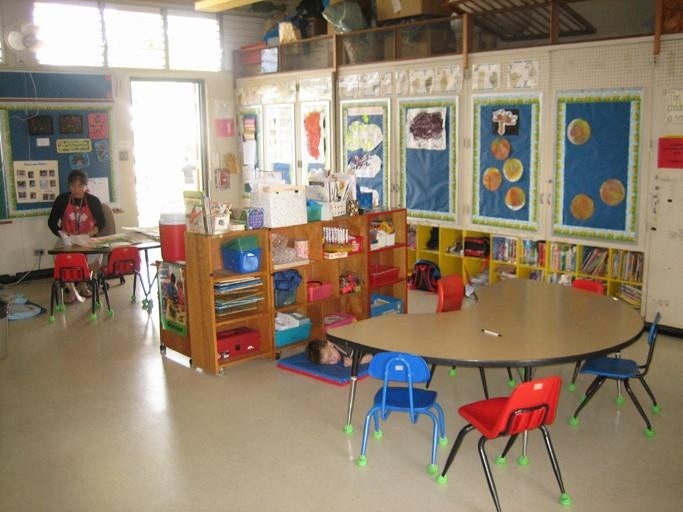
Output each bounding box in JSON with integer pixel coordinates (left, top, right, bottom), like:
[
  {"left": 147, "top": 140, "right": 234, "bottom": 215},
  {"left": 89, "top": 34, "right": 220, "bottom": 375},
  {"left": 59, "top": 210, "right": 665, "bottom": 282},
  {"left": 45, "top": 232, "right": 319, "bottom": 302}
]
[{"left": 4, "top": 20, "right": 44, "bottom": 56}]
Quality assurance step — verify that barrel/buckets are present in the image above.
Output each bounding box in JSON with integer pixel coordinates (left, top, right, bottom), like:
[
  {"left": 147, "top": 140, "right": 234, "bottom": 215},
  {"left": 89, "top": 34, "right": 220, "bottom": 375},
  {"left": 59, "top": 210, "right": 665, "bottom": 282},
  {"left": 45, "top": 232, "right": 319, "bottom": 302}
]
[{"left": 159, "top": 213, "right": 186, "bottom": 262}]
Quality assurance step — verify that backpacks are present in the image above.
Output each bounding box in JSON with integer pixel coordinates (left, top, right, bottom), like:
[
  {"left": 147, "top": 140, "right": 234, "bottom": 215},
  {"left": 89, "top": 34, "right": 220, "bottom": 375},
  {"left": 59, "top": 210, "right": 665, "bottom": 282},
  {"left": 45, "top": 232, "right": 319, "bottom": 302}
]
[
  {"left": 272, "top": 268, "right": 301, "bottom": 293},
  {"left": 409, "top": 259, "right": 440, "bottom": 292},
  {"left": 464, "top": 236, "right": 489, "bottom": 257}
]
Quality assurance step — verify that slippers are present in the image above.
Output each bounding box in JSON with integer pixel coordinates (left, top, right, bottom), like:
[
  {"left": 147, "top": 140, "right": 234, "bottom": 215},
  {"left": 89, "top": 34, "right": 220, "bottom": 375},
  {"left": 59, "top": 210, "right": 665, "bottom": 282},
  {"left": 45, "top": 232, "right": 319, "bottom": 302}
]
[
  {"left": 78, "top": 287, "right": 91, "bottom": 297},
  {"left": 64, "top": 293, "right": 76, "bottom": 304}
]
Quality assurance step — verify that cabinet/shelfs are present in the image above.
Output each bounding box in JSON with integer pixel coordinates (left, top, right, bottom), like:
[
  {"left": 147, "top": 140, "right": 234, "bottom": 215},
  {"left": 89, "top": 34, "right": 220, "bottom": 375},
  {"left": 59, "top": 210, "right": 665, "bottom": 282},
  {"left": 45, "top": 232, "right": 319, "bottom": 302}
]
[
  {"left": 407, "top": 224, "right": 645, "bottom": 314},
  {"left": 185, "top": 206, "right": 408, "bottom": 378}
]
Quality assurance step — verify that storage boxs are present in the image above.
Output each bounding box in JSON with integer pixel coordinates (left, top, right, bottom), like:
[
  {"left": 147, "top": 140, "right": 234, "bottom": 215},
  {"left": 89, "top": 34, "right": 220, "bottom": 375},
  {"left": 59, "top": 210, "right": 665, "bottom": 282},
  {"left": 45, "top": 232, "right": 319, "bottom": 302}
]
[
  {"left": 274, "top": 317, "right": 310, "bottom": 348},
  {"left": 223, "top": 235, "right": 260, "bottom": 251},
  {"left": 240, "top": 0, "right": 456, "bottom": 76},
  {"left": 219, "top": 247, "right": 262, "bottom": 274}
]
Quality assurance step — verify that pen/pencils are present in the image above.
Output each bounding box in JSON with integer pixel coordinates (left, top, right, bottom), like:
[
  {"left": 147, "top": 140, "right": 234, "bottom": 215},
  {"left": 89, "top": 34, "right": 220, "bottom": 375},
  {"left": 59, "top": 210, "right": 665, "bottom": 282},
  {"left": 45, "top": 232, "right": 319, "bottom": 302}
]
[
  {"left": 324, "top": 227, "right": 349, "bottom": 245},
  {"left": 481, "top": 328, "right": 502, "bottom": 338}
]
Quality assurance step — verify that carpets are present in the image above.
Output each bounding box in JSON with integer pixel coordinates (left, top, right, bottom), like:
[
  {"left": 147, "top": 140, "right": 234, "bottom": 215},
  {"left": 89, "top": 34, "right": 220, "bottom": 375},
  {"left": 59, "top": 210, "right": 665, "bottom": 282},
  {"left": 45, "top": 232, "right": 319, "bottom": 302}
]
[{"left": 277, "top": 351, "right": 370, "bottom": 387}]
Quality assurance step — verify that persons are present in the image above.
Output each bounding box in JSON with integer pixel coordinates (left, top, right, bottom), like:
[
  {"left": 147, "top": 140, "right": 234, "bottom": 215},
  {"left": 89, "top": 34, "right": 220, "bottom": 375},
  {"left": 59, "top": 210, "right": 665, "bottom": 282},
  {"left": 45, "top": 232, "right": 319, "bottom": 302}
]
[
  {"left": 304, "top": 333, "right": 383, "bottom": 367},
  {"left": 43, "top": 170, "right": 105, "bottom": 307}
]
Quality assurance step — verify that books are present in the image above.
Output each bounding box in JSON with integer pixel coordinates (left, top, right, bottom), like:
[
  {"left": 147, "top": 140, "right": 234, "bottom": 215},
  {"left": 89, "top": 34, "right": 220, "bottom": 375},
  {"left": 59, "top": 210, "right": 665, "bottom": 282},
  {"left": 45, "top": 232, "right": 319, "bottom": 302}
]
[
  {"left": 210, "top": 269, "right": 265, "bottom": 317},
  {"left": 302, "top": 170, "right": 395, "bottom": 260},
  {"left": 490, "top": 236, "right": 643, "bottom": 313}
]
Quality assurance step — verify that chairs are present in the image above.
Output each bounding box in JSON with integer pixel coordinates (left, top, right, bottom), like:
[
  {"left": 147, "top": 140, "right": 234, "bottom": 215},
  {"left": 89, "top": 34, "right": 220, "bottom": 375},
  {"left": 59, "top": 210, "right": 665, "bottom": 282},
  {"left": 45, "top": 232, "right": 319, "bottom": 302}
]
[
  {"left": 436, "top": 376, "right": 572, "bottom": 512},
  {"left": 569, "top": 312, "right": 662, "bottom": 436},
  {"left": 48, "top": 253, "right": 114, "bottom": 323},
  {"left": 425, "top": 274, "right": 515, "bottom": 399},
  {"left": 572, "top": 280, "right": 605, "bottom": 295},
  {"left": 96, "top": 204, "right": 115, "bottom": 237},
  {"left": 356, "top": 352, "right": 448, "bottom": 475},
  {"left": 95, "top": 246, "right": 147, "bottom": 317}
]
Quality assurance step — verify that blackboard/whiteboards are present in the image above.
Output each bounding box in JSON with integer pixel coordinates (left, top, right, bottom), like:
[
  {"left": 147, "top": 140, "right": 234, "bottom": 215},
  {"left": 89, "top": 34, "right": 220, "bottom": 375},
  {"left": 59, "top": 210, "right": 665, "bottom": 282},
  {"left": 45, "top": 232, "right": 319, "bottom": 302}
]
[{"left": 1, "top": 74, "right": 117, "bottom": 222}]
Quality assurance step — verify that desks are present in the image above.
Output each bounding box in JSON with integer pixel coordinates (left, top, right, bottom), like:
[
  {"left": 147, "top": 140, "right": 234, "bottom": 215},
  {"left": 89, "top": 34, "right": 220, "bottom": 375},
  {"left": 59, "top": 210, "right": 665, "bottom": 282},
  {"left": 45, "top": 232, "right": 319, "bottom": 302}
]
[
  {"left": 328, "top": 278, "right": 644, "bottom": 465},
  {"left": 48, "top": 226, "right": 161, "bottom": 292}
]
